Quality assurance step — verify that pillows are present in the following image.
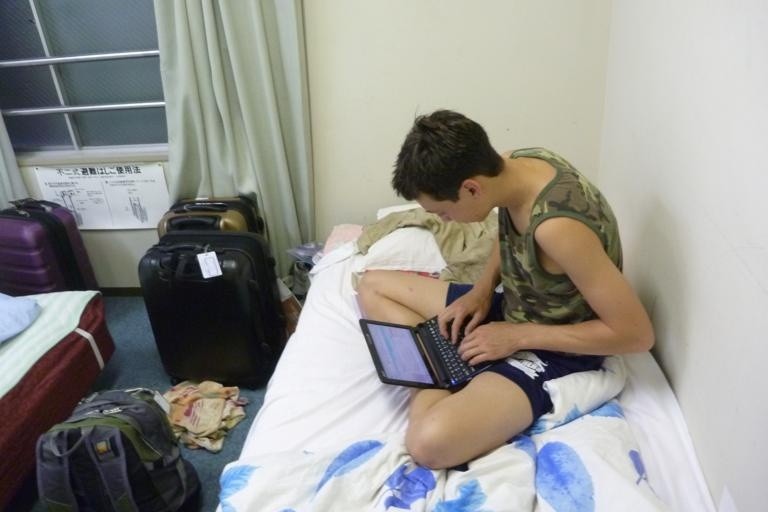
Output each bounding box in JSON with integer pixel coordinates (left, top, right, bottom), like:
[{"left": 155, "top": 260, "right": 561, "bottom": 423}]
[{"left": 356, "top": 200, "right": 500, "bottom": 280}]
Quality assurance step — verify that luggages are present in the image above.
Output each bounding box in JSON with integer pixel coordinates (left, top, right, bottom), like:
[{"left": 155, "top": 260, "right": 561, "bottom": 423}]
[
  {"left": 0, "top": 198, "right": 98, "bottom": 296},
  {"left": 139, "top": 230, "right": 289, "bottom": 389},
  {"left": 157, "top": 192, "right": 264, "bottom": 241}
]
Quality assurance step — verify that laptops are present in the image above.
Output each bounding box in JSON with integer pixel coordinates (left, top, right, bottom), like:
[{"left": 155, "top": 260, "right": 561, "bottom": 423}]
[{"left": 359, "top": 314, "right": 494, "bottom": 389}]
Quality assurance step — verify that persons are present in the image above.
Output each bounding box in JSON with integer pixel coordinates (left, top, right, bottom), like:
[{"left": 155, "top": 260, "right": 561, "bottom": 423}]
[{"left": 357, "top": 107, "right": 655, "bottom": 469}]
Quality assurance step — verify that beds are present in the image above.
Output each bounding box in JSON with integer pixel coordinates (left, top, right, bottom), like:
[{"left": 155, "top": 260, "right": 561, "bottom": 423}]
[{"left": 218, "top": 223, "right": 716, "bottom": 512}]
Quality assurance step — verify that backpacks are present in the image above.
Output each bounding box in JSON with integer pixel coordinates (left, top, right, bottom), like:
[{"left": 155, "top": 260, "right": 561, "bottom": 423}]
[{"left": 36, "top": 387, "right": 202, "bottom": 512}]
